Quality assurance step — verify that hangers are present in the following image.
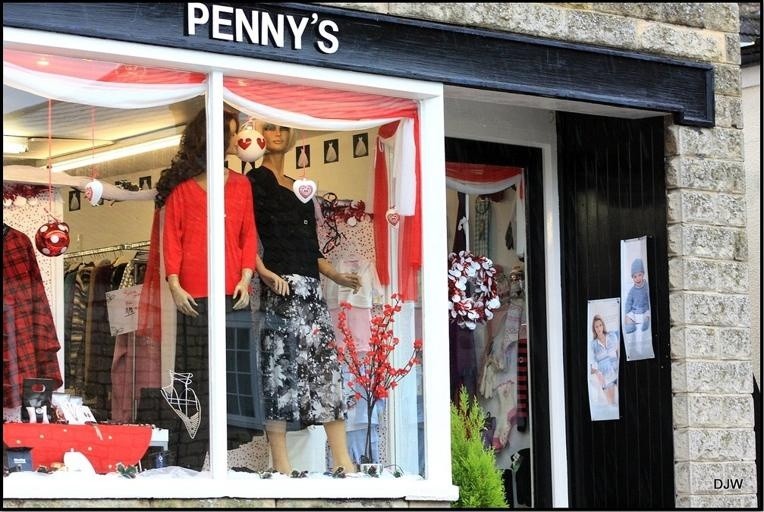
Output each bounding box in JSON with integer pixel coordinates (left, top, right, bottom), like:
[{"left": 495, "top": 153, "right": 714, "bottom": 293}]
[
  {"left": 64, "top": 240, "right": 150, "bottom": 283},
  {"left": 321, "top": 192, "right": 347, "bottom": 254},
  {"left": 141, "top": 371, "right": 207, "bottom": 412}
]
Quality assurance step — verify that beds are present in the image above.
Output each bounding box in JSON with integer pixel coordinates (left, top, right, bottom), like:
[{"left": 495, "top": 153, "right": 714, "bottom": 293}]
[{"left": 3, "top": 423, "right": 168, "bottom": 472}]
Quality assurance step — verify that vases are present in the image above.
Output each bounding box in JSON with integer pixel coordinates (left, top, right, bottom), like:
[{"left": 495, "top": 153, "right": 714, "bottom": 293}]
[{"left": 361, "top": 463, "right": 383, "bottom": 478}]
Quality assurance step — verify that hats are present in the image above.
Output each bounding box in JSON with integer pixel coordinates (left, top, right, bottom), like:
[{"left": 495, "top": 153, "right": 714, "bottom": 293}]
[{"left": 632, "top": 259, "right": 644, "bottom": 277}]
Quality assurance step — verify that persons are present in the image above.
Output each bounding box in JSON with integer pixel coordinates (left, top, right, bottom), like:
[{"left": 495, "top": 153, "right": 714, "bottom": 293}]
[
  {"left": 155, "top": 109, "right": 258, "bottom": 472},
  {"left": 589, "top": 316, "right": 620, "bottom": 406},
  {"left": 248, "top": 116, "right": 363, "bottom": 476},
  {"left": 623, "top": 259, "right": 651, "bottom": 333}
]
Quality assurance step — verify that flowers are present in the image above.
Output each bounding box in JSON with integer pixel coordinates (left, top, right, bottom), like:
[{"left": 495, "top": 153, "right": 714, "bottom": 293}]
[
  {"left": 111, "top": 179, "right": 139, "bottom": 206},
  {"left": 448, "top": 250, "right": 501, "bottom": 330},
  {"left": 314, "top": 294, "right": 423, "bottom": 463}
]
